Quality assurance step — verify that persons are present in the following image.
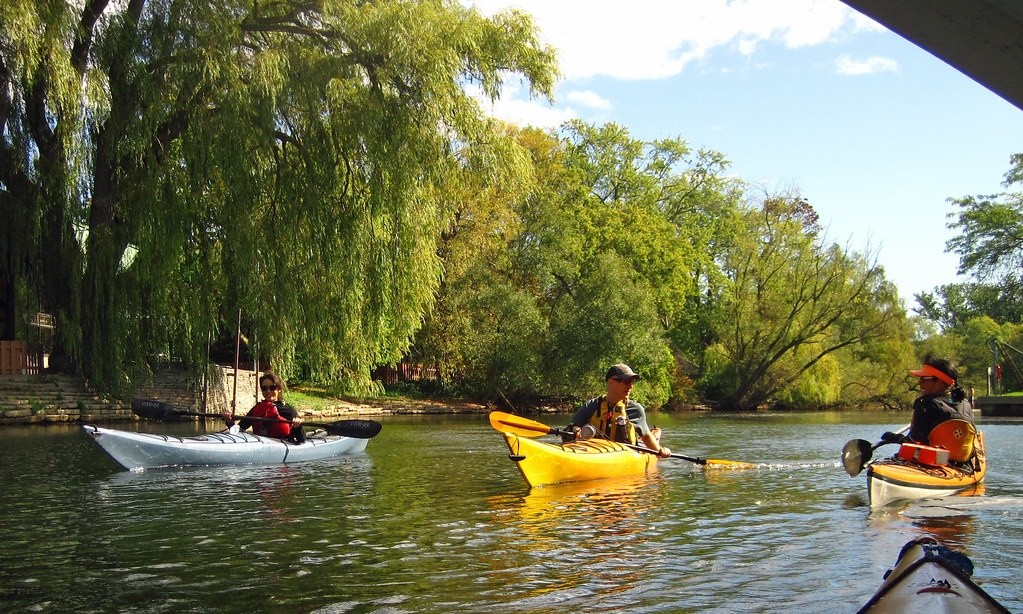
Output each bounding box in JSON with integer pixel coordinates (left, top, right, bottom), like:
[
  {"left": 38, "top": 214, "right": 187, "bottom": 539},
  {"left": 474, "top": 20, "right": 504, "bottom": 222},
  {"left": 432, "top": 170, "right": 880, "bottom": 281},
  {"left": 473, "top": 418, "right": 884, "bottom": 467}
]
[
  {"left": 223, "top": 371, "right": 307, "bottom": 443},
  {"left": 567, "top": 364, "right": 672, "bottom": 458},
  {"left": 881, "top": 358, "right": 974, "bottom": 444}
]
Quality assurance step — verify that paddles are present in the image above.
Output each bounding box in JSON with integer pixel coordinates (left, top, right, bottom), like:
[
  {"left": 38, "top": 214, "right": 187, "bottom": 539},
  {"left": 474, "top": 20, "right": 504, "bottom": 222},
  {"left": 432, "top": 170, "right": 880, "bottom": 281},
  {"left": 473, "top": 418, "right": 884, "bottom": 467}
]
[
  {"left": 488, "top": 411, "right": 756, "bottom": 472},
  {"left": 130, "top": 397, "right": 382, "bottom": 441},
  {"left": 841, "top": 421, "right": 913, "bottom": 479}
]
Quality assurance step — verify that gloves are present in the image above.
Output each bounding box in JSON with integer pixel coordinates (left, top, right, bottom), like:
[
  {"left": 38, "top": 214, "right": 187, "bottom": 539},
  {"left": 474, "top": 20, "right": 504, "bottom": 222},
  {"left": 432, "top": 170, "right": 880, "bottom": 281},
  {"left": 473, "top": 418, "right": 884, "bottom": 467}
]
[{"left": 881, "top": 431, "right": 904, "bottom": 442}]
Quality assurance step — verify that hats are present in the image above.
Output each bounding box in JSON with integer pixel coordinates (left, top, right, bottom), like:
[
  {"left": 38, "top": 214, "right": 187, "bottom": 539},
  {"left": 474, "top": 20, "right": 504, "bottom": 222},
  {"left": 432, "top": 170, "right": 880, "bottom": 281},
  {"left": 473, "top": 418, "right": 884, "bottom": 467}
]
[
  {"left": 606, "top": 363, "right": 639, "bottom": 383},
  {"left": 909, "top": 364, "right": 954, "bottom": 386}
]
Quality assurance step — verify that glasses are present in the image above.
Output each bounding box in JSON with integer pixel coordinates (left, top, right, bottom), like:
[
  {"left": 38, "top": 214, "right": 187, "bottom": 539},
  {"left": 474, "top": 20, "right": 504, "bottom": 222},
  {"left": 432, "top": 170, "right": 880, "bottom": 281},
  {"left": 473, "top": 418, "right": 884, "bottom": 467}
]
[
  {"left": 261, "top": 385, "right": 278, "bottom": 391},
  {"left": 611, "top": 377, "right": 633, "bottom": 383},
  {"left": 917, "top": 376, "right": 934, "bottom": 383}
]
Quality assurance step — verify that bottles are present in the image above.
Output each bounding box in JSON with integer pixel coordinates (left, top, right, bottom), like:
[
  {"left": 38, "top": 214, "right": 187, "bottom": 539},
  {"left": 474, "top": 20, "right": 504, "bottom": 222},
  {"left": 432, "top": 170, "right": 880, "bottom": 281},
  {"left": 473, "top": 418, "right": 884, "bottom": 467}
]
[
  {"left": 616, "top": 416, "right": 628, "bottom": 440},
  {"left": 229, "top": 424, "right": 240, "bottom": 435}
]
